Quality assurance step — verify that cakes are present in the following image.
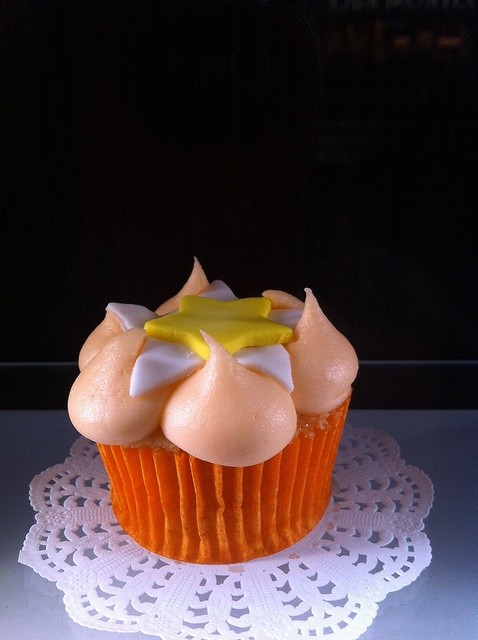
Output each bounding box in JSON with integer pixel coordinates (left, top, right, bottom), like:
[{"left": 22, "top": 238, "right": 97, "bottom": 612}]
[{"left": 65, "top": 254, "right": 361, "bottom": 566}]
